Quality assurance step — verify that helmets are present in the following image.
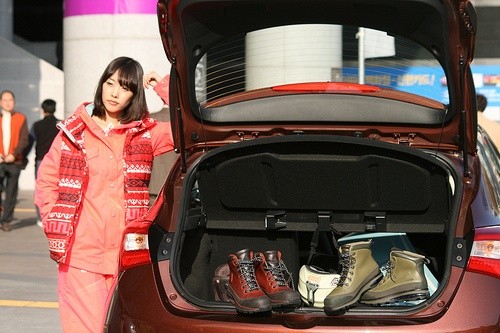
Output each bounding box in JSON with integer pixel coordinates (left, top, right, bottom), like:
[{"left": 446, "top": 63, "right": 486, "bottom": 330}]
[{"left": 298, "top": 264, "right": 342, "bottom": 308}]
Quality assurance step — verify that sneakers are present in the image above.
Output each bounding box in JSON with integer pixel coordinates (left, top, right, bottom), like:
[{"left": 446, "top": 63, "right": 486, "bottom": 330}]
[
  {"left": 2, "top": 221, "right": 10, "bottom": 230},
  {"left": 227, "top": 248, "right": 272, "bottom": 313},
  {"left": 360, "top": 246, "right": 431, "bottom": 306},
  {"left": 323, "top": 238, "right": 383, "bottom": 315},
  {"left": 254, "top": 249, "right": 301, "bottom": 308}
]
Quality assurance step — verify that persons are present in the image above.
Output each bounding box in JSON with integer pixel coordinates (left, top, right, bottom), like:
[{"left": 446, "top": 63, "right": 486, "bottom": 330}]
[
  {"left": 35, "top": 57, "right": 175, "bottom": 333},
  {"left": 0, "top": 90, "right": 29, "bottom": 230},
  {"left": 24, "top": 99, "right": 63, "bottom": 228},
  {"left": 475, "top": 95, "right": 500, "bottom": 151}
]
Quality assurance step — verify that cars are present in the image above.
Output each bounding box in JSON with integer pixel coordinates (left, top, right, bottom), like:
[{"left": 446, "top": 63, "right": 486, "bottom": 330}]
[{"left": 104, "top": 0, "right": 500, "bottom": 333}]
